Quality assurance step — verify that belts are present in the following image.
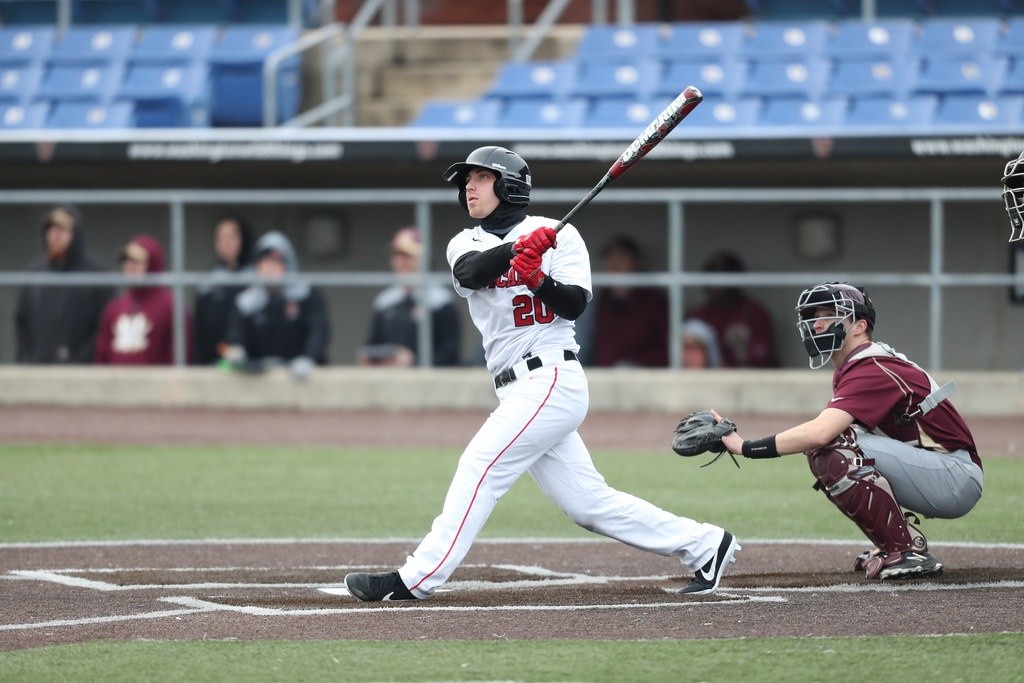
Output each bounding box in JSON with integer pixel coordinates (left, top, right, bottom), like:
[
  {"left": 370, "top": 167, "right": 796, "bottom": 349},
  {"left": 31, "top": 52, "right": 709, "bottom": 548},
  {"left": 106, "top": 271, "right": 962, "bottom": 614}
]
[{"left": 494, "top": 349, "right": 579, "bottom": 389}]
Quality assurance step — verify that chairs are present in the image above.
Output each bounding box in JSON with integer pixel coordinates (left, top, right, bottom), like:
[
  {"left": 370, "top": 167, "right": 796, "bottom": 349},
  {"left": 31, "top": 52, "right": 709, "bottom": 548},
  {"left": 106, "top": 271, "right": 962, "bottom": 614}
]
[
  {"left": 0, "top": 0, "right": 319, "bottom": 128},
  {"left": 410, "top": 1, "right": 1024, "bottom": 130}
]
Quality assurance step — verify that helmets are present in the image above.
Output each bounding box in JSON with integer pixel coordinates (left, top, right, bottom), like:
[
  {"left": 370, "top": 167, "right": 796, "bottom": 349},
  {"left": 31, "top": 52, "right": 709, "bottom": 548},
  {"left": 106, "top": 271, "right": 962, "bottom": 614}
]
[
  {"left": 441, "top": 147, "right": 532, "bottom": 210},
  {"left": 1000, "top": 150, "right": 1024, "bottom": 242},
  {"left": 795, "top": 281, "right": 876, "bottom": 370}
]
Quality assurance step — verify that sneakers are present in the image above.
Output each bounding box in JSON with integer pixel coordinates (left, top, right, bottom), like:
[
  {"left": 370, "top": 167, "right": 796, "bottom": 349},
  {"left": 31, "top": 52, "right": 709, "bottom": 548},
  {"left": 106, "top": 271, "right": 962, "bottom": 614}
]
[
  {"left": 853, "top": 549, "right": 888, "bottom": 570},
  {"left": 879, "top": 548, "right": 944, "bottom": 582},
  {"left": 673, "top": 530, "right": 743, "bottom": 594},
  {"left": 342, "top": 570, "right": 422, "bottom": 602}
]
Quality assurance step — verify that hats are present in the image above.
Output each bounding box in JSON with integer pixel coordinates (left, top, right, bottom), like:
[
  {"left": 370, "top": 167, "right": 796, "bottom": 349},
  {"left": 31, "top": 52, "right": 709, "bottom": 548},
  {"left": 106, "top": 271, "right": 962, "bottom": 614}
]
[
  {"left": 46, "top": 209, "right": 76, "bottom": 230},
  {"left": 596, "top": 237, "right": 640, "bottom": 262},
  {"left": 120, "top": 240, "right": 150, "bottom": 263}
]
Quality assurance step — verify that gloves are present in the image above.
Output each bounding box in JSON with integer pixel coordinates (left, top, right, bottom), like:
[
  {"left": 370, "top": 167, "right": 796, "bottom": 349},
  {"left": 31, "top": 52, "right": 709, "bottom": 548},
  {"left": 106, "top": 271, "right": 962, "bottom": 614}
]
[
  {"left": 510, "top": 227, "right": 559, "bottom": 256},
  {"left": 509, "top": 250, "right": 547, "bottom": 291}
]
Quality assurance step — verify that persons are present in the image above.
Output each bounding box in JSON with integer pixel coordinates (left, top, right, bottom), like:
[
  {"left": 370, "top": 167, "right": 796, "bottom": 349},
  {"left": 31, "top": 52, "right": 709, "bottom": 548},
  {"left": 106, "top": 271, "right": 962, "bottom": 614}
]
[
  {"left": 672, "top": 282, "right": 983, "bottom": 580},
  {"left": 363, "top": 228, "right": 461, "bottom": 367},
  {"left": 345, "top": 146, "right": 742, "bottom": 601},
  {"left": 14, "top": 207, "right": 113, "bottom": 363},
  {"left": 96, "top": 234, "right": 172, "bottom": 363},
  {"left": 190, "top": 217, "right": 330, "bottom": 369},
  {"left": 591, "top": 237, "right": 774, "bottom": 367},
  {"left": 1000, "top": 150, "right": 1024, "bottom": 242}
]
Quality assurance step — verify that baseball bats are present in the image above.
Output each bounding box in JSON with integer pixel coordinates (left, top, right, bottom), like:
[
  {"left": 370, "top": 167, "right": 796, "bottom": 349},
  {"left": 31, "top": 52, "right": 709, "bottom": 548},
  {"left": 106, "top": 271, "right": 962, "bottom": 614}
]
[{"left": 554, "top": 86, "right": 704, "bottom": 233}]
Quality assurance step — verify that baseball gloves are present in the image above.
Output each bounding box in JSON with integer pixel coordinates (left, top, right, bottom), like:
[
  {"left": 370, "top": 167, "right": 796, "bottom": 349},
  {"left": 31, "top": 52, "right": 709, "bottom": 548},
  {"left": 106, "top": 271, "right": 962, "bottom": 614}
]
[{"left": 671, "top": 410, "right": 736, "bottom": 456}]
